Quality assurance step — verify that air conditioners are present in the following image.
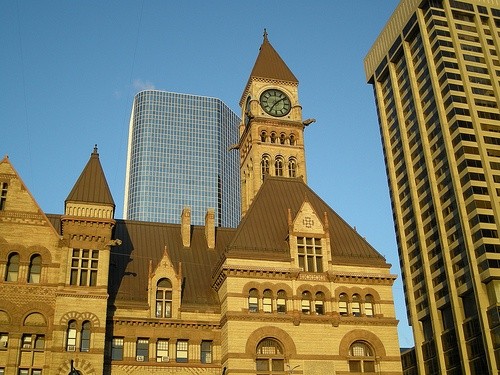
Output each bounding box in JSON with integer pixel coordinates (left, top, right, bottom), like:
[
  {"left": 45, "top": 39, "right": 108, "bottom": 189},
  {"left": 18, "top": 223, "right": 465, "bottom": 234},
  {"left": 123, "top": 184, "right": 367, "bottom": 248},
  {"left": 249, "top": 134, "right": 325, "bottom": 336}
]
[
  {"left": 68, "top": 345, "right": 75, "bottom": 351},
  {"left": 162, "top": 356, "right": 170, "bottom": 363},
  {"left": 136, "top": 355, "right": 144, "bottom": 361}
]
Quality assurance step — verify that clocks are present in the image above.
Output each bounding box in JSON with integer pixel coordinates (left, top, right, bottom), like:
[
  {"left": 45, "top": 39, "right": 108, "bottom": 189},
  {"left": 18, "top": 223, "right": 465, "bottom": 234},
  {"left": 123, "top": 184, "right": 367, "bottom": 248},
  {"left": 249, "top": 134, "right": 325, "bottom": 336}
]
[
  {"left": 243, "top": 95, "right": 253, "bottom": 128},
  {"left": 260, "top": 88, "right": 291, "bottom": 117}
]
